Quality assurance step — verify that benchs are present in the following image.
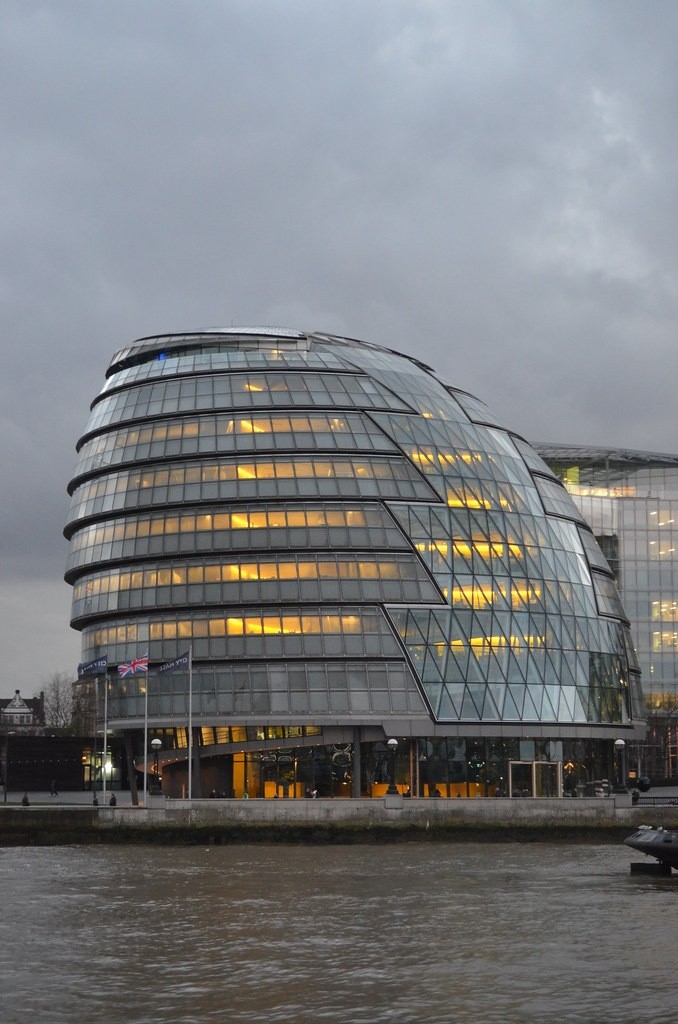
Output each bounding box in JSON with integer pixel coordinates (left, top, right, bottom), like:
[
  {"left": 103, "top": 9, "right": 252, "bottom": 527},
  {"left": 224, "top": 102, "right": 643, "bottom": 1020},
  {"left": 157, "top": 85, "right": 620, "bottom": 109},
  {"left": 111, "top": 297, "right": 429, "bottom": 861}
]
[{"left": 612, "top": 783, "right": 629, "bottom": 794}]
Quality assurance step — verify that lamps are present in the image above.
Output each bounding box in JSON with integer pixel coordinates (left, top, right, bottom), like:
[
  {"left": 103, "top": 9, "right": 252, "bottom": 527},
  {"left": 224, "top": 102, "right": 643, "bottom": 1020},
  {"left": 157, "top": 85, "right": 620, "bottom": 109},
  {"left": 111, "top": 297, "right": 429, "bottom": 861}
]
[{"left": 627, "top": 768, "right": 638, "bottom": 780}]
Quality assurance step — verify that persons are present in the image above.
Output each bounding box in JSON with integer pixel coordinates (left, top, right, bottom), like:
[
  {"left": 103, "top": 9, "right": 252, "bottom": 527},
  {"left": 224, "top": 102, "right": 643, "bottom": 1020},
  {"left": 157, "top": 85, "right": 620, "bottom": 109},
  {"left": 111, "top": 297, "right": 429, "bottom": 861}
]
[
  {"left": 110, "top": 794, "right": 117, "bottom": 806},
  {"left": 50, "top": 779, "right": 59, "bottom": 797},
  {"left": 632, "top": 789, "right": 640, "bottom": 805},
  {"left": 93, "top": 792, "right": 99, "bottom": 806},
  {"left": 242, "top": 791, "right": 249, "bottom": 799},
  {"left": 434, "top": 789, "right": 441, "bottom": 797},
  {"left": 457, "top": 791, "right": 461, "bottom": 797},
  {"left": 496, "top": 785, "right": 532, "bottom": 798},
  {"left": 571, "top": 787, "right": 578, "bottom": 797},
  {"left": 274, "top": 791, "right": 278, "bottom": 798},
  {"left": 210, "top": 787, "right": 235, "bottom": 798},
  {"left": 407, "top": 789, "right": 411, "bottom": 796},
  {"left": 306, "top": 788, "right": 318, "bottom": 799},
  {"left": 22, "top": 793, "right": 30, "bottom": 806}
]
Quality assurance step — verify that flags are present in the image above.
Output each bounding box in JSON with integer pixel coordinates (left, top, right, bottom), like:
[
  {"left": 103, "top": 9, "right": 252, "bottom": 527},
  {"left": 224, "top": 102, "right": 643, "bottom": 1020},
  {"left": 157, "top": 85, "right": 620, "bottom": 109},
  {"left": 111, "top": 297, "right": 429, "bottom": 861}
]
[
  {"left": 156, "top": 650, "right": 191, "bottom": 678},
  {"left": 78, "top": 655, "right": 107, "bottom": 680},
  {"left": 117, "top": 654, "right": 150, "bottom": 679}
]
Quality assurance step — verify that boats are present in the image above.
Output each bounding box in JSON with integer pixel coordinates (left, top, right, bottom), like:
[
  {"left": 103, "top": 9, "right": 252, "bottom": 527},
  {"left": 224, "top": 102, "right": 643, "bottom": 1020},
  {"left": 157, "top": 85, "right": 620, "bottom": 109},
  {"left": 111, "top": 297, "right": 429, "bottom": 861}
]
[{"left": 623, "top": 824, "right": 678, "bottom": 873}]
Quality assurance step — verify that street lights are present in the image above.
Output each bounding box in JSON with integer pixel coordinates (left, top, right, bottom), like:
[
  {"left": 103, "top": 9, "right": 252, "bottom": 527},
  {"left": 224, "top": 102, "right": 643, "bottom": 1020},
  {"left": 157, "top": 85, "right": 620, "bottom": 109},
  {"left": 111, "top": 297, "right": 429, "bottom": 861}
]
[
  {"left": 387, "top": 739, "right": 399, "bottom": 795},
  {"left": 614, "top": 738, "right": 627, "bottom": 793},
  {"left": 151, "top": 739, "right": 164, "bottom": 796},
  {"left": 92, "top": 729, "right": 114, "bottom": 806}
]
